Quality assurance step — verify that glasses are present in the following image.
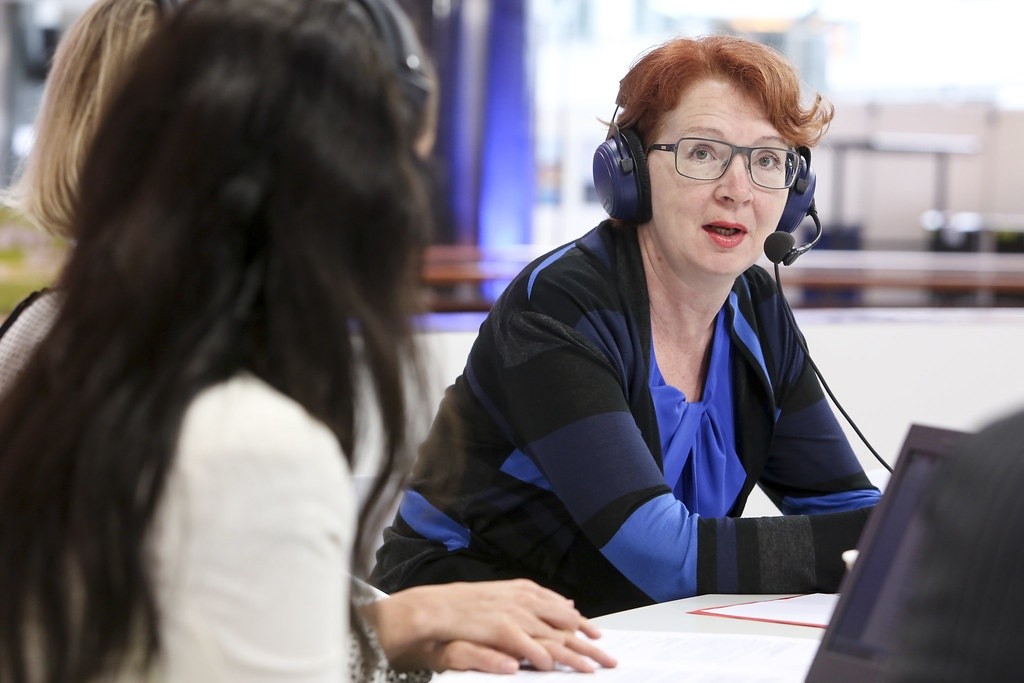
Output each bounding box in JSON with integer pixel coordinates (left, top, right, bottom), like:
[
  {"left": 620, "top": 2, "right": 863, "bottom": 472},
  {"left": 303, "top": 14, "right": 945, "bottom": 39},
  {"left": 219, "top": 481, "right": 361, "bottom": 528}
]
[{"left": 647, "top": 137, "right": 801, "bottom": 190}]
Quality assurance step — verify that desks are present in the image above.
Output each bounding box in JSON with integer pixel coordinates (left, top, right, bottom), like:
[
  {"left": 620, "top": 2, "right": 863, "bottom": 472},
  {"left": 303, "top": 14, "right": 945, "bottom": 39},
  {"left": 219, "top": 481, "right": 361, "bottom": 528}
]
[{"left": 587, "top": 593, "right": 826, "bottom": 683}]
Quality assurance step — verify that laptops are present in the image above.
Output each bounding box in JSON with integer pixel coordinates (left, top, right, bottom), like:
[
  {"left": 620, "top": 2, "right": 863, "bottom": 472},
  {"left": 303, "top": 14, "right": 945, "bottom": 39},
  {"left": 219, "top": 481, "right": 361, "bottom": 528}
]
[{"left": 807, "top": 423, "right": 973, "bottom": 683}]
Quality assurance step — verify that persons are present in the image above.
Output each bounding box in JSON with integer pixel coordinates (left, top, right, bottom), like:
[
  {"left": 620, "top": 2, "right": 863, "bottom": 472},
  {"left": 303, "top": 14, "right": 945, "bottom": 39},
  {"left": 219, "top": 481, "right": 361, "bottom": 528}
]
[
  {"left": 0, "top": 1, "right": 617, "bottom": 683},
  {"left": 369, "top": 32, "right": 890, "bottom": 618}
]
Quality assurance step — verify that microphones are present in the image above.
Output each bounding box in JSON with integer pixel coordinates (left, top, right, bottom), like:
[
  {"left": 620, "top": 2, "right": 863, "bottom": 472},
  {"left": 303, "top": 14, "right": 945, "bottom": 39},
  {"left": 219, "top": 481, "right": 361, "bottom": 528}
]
[{"left": 762, "top": 211, "right": 823, "bottom": 265}]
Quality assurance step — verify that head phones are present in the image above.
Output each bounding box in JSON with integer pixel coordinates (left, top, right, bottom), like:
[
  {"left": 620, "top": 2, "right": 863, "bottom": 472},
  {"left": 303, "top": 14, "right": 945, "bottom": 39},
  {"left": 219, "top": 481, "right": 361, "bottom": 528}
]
[{"left": 592, "top": 98, "right": 817, "bottom": 234}]
